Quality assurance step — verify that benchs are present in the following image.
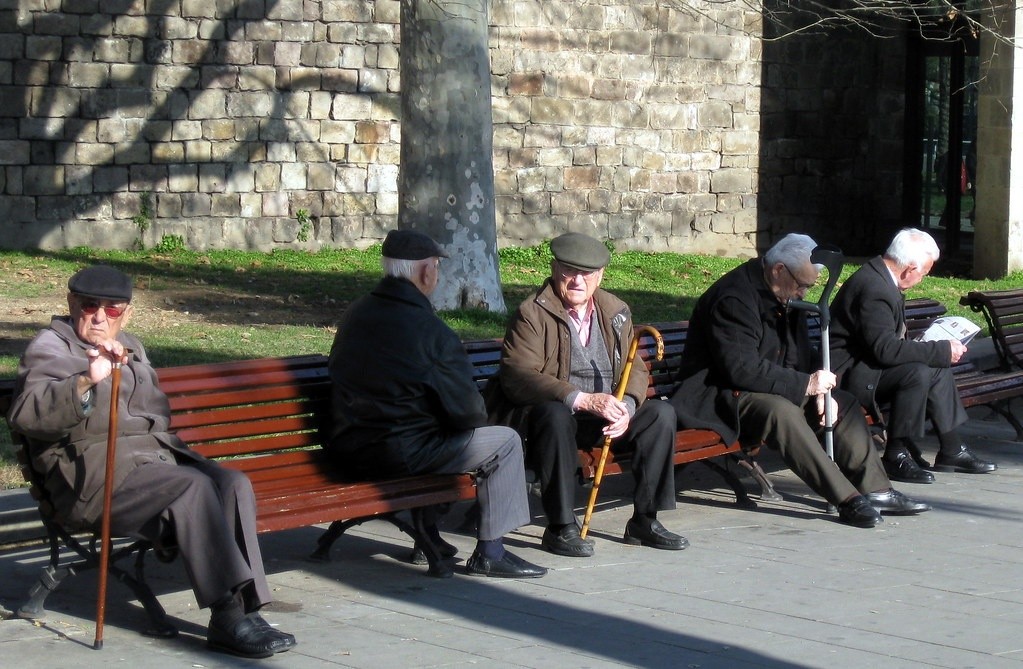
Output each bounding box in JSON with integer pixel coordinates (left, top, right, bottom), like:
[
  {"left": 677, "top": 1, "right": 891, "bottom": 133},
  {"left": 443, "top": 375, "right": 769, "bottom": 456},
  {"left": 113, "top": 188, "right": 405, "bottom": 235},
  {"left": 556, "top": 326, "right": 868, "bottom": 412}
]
[{"left": 0, "top": 289, "right": 1022, "bottom": 640}]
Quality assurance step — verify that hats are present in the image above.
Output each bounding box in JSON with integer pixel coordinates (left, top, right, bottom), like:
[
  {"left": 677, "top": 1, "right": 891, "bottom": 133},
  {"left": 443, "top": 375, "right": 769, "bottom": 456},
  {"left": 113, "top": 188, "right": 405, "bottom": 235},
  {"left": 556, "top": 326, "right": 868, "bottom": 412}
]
[
  {"left": 381, "top": 229, "right": 451, "bottom": 261},
  {"left": 68, "top": 265, "right": 131, "bottom": 300},
  {"left": 550, "top": 232, "right": 610, "bottom": 273}
]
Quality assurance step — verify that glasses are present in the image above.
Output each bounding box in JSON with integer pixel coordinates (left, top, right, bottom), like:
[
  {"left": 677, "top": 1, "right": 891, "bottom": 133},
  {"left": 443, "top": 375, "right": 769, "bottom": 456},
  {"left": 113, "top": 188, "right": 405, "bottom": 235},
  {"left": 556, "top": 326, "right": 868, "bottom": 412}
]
[
  {"left": 75, "top": 294, "right": 130, "bottom": 319},
  {"left": 783, "top": 265, "right": 815, "bottom": 292}
]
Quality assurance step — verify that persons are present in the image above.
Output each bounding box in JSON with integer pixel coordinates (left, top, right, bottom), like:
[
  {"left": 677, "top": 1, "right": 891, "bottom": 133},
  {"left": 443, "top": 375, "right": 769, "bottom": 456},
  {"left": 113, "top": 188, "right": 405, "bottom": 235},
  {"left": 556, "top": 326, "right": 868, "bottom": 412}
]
[
  {"left": 8, "top": 266, "right": 295, "bottom": 657},
  {"left": 818, "top": 229, "right": 998, "bottom": 483},
  {"left": 670, "top": 234, "right": 930, "bottom": 527},
  {"left": 484, "top": 233, "right": 690, "bottom": 557},
  {"left": 328, "top": 230, "right": 547, "bottom": 578}
]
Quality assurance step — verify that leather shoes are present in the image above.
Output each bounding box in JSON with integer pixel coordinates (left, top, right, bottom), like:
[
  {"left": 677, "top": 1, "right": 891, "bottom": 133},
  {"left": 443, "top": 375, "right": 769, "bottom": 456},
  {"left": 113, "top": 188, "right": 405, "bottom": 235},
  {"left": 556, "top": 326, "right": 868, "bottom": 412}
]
[
  {"left": 411, "top": 543, "right": 428, "bottom": 563},
  {"left": 624, "top": 518, "right": 688, "bottom": 550},
  {"left": 467, "top": 550, "right": 548, "bottom": 579},
  {"left": 541, "top": 523, "right": 593, "bottom": 557},
  {"left": 251, "top": 615, "right": 296, "bottom": 649},
  {"left": 934, "top": 443, "right": 998, "bottom": 472},
  {"left": 881, "top": 454, "right": 935, "bottom": 484},
  {"left": 839, "top": 496, "right": 883, "bottom": 527},
  {"left": 206, "top": 617, "right": 286, "bottom": 656},
  {"left": 865, "top": 488, "right": 930, "bottom": 514}
]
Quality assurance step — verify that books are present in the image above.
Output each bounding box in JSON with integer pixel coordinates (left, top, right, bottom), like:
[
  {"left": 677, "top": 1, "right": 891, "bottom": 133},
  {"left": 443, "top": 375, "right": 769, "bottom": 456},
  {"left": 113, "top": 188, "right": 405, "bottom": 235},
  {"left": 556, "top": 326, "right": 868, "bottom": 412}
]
[{"left": 912, "top": 317, "right": 985, "bottom": 346}]
